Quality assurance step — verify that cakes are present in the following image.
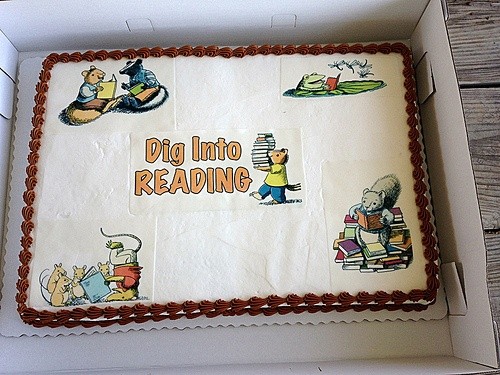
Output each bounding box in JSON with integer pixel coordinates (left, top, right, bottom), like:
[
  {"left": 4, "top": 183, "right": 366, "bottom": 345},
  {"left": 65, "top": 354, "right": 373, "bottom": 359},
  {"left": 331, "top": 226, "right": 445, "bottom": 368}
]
[{"left": 16, "top": 42, "right": 441, "bottom": 329}]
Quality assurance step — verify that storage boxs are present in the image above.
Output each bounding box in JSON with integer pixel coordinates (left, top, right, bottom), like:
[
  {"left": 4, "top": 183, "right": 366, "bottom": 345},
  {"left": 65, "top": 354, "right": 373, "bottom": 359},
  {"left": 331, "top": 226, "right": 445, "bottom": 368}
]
[{"left": 0, "top": 0, "right": 500, "bottom": 375}]
